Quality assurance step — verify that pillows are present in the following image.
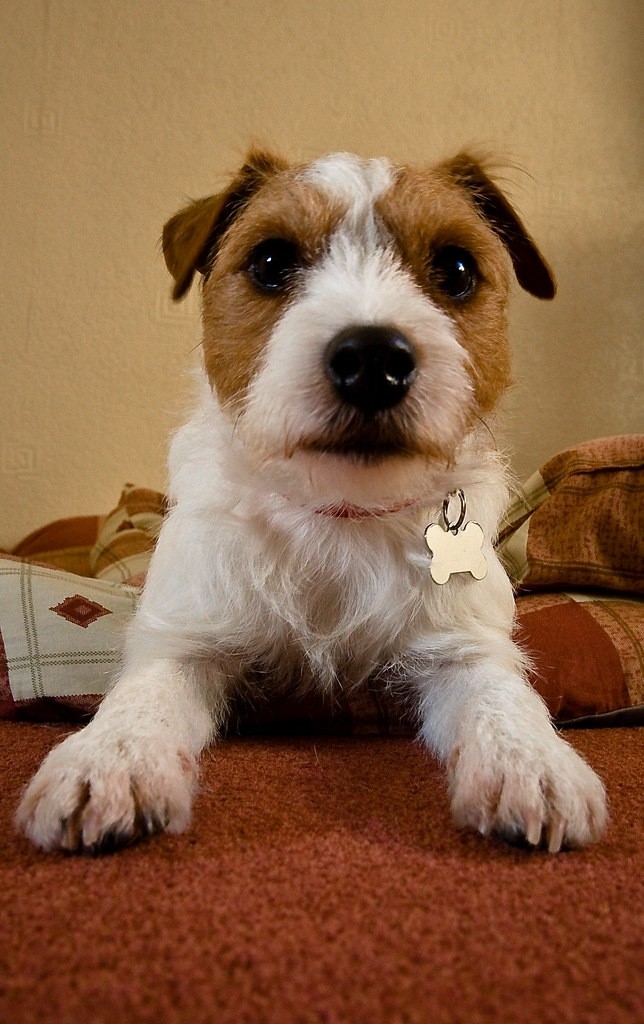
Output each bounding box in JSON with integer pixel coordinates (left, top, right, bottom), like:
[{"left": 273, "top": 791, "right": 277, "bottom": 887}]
[
  {"left": 0, "top": 550, "right": 644, "bottom": 730},
  {"left": 80, "top": 433, "right": 644, "bottom": 601}
]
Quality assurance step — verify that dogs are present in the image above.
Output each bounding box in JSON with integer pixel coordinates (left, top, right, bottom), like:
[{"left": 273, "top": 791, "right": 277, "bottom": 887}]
[{"left": 12, "top": 142, "right": 613, "bottom": 853}]
